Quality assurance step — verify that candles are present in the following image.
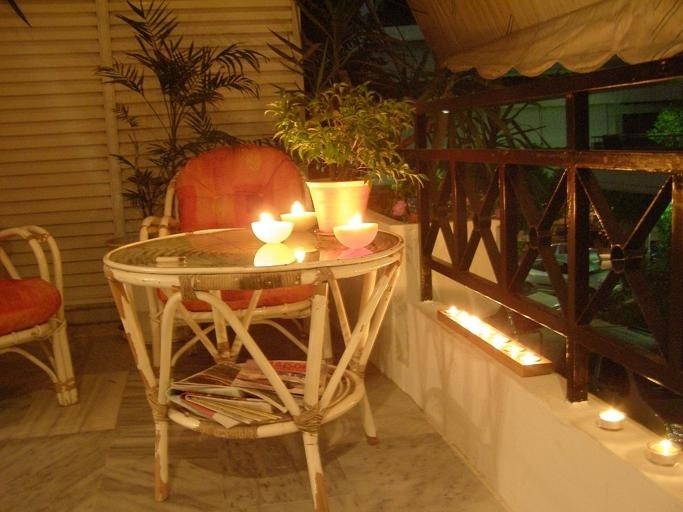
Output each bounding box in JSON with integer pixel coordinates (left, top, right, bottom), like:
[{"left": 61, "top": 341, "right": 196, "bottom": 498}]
[
  {"left": 285, "top": 236, "right": 318, "bottom": 263},
  {"left": 253, "top": 243, "right": 293, "bottom": 267},
  {"left": 446, "top": 305, "right": 541, "bottom": 364},
  {"left": 598, "top": 408, "right": 626, "bottom": 431},
  {"left": 646, "top": 438, "right": 680, "bottom": 465},
  {"left": 252, "top": 212, "right": 294, "bottom": 244},
  {"left": 279, "top": 201, "right": 316, "bottom": 233},
  {"left": 334, "top": 213, "right": 379, "bottom": 247}
]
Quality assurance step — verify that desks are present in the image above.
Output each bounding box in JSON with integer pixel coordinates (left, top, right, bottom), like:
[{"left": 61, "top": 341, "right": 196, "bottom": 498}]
[{"left": 103, "top": 218, "right": 405, "bottom": 512}]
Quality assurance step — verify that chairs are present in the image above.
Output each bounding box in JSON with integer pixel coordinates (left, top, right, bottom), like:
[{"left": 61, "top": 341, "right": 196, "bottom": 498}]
[
  {"left": 0, "top": 221, "right": 78, "bottom": 408},
  {"left": 140, "top": 145, "right": 332, "bottom": 382}
]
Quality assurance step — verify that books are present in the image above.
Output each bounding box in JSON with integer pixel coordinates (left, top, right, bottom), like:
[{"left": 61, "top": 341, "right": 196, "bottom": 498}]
[{"left": 166, "top": 360, "right": 327, "bottom": 428}]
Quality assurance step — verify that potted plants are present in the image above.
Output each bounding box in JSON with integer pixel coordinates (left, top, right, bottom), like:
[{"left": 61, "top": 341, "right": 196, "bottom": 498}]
[
  {"left": 93, "top": 1, "right": 270, "bottom": 345},
  {"left": 265, "top": 79, "right": 429, "bottom": 238}
]
